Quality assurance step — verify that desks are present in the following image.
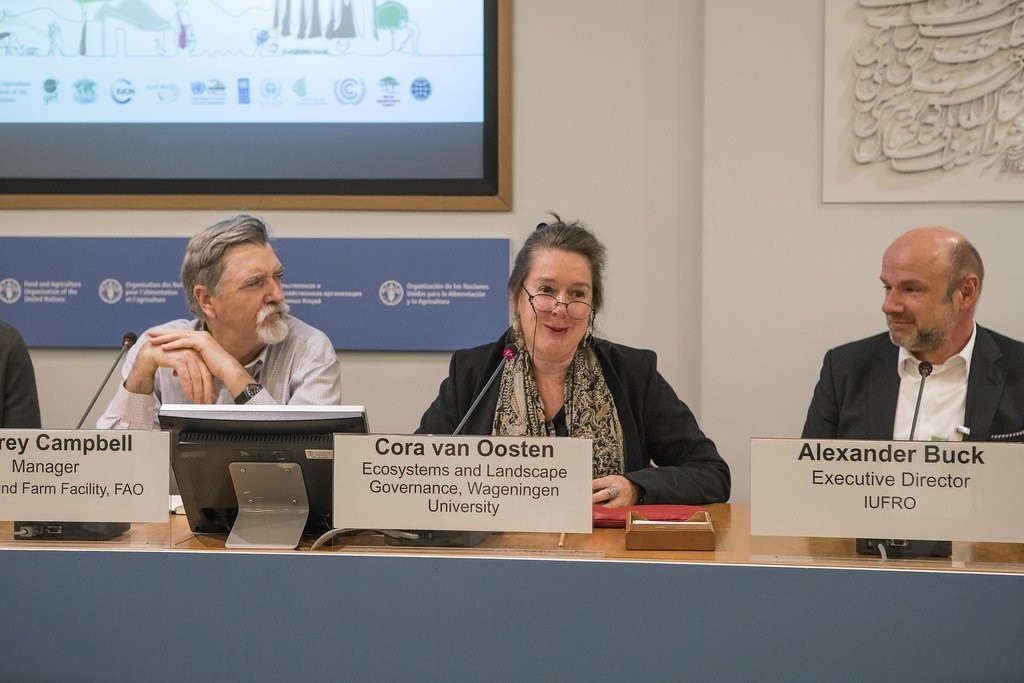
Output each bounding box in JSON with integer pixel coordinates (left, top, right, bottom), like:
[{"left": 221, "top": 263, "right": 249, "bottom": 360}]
[{"left": 1, "top": 497, "right": 1024, "bottom": 683}]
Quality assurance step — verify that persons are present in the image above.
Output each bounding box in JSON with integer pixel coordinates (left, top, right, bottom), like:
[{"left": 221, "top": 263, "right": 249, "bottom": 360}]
[
  {"left": 413, "top": 222, "right": 732, "bottom": 508},
  {"left": 801, "top": 227, "right": 1024, "bottom": 443},
  {"left": 0, "top": 320, "right": 42, "bottom": 429},
  {"left": 97, "top": 215, "right": 342, "bottom": 495}
]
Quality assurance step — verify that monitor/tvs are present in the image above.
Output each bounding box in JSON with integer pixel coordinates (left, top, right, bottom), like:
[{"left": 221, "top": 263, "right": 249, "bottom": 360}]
[{"left": 158, "top": 403, "right": 367, "bottom": 548}]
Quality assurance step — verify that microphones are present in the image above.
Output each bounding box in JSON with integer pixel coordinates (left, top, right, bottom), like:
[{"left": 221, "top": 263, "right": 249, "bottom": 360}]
[
  {"left": 76, "top": 332, "right": 137, "bottom": 430},
  {"left": 909, "top": 360, "right": 933, "bottom": 440},
  {"left": 453, "top": 342, "right": 518, "bottom": 434}
]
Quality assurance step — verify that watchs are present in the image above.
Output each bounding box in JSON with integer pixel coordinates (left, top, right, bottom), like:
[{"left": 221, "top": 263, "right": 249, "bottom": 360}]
[{"left": 234, "top": 383, "right": 263, "bottom": 405}]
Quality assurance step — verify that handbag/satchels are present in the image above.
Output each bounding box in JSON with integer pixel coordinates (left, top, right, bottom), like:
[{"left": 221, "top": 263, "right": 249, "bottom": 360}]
[{"left": 593, "top": 503, "right": 709, "bottom": 526}]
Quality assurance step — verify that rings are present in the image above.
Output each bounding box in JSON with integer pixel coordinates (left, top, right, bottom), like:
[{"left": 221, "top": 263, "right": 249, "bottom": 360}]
[
  {"left": 608, "top": 486, "right": 620, "bottom": 499},
  {"left": 187, "top": 332, "right": 190, "bottom": 337}
]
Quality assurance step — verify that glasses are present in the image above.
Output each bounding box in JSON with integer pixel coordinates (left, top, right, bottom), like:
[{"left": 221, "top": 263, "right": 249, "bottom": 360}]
[{"left": 521, "top": 282, "right": 597, "bottom": 320}]
